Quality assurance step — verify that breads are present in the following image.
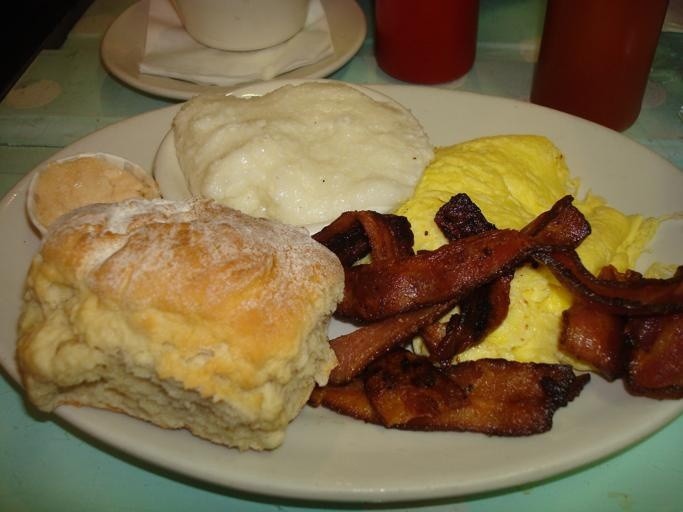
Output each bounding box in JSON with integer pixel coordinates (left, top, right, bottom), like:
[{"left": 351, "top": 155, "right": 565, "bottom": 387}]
[{"left": 16, "top": 196, "right": 344, "bottom": 452}]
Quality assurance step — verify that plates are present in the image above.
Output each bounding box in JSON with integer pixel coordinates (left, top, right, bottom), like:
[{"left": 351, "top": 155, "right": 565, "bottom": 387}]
[
  {"left": 0, "top": 81, "right": 682, "bottom": 505},
  {"left": 99, "top": 0, "right": 369, "bottom": 103}
]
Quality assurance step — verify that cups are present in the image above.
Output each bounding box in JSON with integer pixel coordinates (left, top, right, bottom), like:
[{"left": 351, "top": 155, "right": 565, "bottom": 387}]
[
  {"left": 170, "top": 0, "right": 309, "bottom": 54},
  {"left": 371, "top": 0, "right": 477, "bottom": 83},
  {"left": 530, "top": 0, "right": 671, "bottom": 134}
]
